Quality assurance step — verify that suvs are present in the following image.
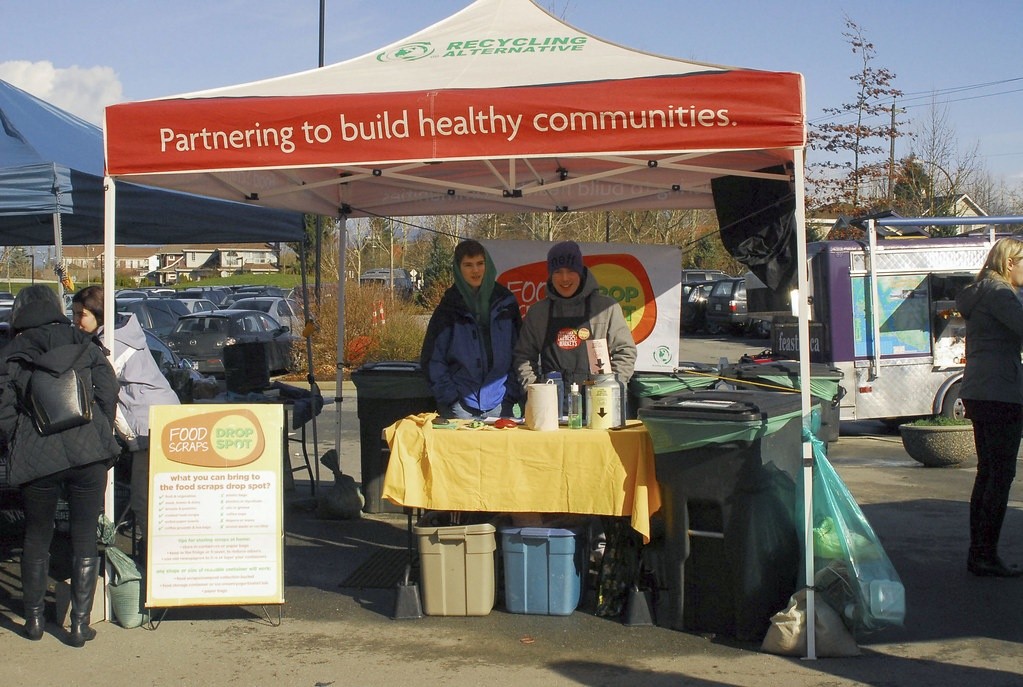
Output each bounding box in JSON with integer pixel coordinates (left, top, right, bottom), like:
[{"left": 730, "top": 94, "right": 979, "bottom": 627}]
[
  {"left": 237, "top": 285, "right": 283, "bottom": 297},
  {"left": 114, "top": 293, "right": 198, "bottom": 342},
  {"left": 682, "top": 270, "right": 734, "bottom": 281}
]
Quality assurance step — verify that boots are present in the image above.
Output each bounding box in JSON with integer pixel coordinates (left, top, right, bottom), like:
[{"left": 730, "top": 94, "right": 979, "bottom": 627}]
[
  {"left": 70, "top": 556, "right": 100, "bottom": 648},
  {"left": 20, "top": 550, "right": 49, "bottom": 640}
]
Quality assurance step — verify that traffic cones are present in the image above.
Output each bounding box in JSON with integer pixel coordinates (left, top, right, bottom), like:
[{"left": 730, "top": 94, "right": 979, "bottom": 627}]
[
  {"left": 371, "top": 301, "right": 378, "bottom": 335},
  {"left": 379, "top": 302, "right": 387, "bottom": 339}
]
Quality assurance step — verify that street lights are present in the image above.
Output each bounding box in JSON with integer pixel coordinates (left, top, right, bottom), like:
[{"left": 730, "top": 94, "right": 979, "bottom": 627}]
[{"left": 912, "top": 159, "right": 957, "bottom": 218}]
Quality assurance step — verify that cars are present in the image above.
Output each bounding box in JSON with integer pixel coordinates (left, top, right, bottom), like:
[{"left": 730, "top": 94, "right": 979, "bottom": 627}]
[
  {"left": 681, "top": 280, "right": 719, "bottom": 335},
  {"left": 0, "top": 308, "right": 12, "bottom": 332},
  {"left": 175, "top": 298, "right": 220, "bottom": 314},
  {"left": 217, "top": 292, "right": 267, "bottom": 310},
  {"left": 142, "top": 330, "right": 204, "bottom": 386},
  {"left": 225, "top": 297, "right": 312, "bottom": 335},
  {"left": 166, "top": 310, "right": 300, "bottom": 380},
  {"left": 115, "top": 285, "right": 248, "bottom": 304},
  {"left": 0, "top": 291, "right": 16, "bottom": 307}
]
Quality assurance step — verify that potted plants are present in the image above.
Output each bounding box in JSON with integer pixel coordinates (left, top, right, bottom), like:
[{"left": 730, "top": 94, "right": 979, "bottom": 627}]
[{"left": 899, "top": 416, "right": 975, "bottom": 465}]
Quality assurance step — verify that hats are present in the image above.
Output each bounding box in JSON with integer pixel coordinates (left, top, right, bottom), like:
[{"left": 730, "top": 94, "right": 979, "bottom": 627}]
[{"left": 548, "top": 238, "right": 584, "bottom": 280}]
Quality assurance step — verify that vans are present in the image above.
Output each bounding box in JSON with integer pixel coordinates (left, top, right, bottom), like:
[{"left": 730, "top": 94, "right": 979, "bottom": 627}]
[{"left": 706, "top": 278, "right": 793, "bottom": 338}]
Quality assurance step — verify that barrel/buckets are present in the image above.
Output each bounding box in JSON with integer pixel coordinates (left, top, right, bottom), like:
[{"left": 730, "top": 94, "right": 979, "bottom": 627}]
[{"left": 584, "top": 371, "right": 628, "bottom": 430}]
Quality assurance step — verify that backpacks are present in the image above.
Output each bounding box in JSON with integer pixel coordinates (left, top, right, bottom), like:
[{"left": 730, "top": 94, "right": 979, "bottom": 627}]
[{"left": 11, "top": 356, "right": 95, "bottom": 433}]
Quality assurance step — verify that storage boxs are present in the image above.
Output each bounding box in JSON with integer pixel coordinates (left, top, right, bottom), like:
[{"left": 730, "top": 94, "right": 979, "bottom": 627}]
[{"left": 408, "top": 506, "right": 589, "bottom": 617}]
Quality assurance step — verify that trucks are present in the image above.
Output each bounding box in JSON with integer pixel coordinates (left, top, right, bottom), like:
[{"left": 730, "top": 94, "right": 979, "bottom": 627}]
[{"left": 807, "top": 216, "right": 1023, "bottom": 421}]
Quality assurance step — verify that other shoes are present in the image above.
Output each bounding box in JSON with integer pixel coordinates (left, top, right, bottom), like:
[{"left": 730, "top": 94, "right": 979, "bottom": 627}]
[{"left": 968, "top": 555, "right": 1023, "bottom": 577}]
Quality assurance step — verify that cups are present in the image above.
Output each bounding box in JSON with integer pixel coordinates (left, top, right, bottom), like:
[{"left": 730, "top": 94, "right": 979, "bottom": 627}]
[{"left": 548, "top": 371, "right": 564, "bottom": 421}]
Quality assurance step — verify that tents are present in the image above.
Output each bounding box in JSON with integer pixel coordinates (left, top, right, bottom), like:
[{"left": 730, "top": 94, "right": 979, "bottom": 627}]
[
  {"left": 0, "top": 78, "right": 321, "bottom": 497},
  {"left": 104, "top": 0, "right": 817, "bottom": 659}
]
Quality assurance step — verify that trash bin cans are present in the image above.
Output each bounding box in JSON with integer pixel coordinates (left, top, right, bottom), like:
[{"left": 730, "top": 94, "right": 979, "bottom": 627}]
[
  {"left": 360, "top": 268, "right": 412, "bottom": 302},
  {"left": 630, "top": 357, "right": 846, "bottom": 646},
  {"left": 351, "top": 363, "right": 437, "bottom": 512}
]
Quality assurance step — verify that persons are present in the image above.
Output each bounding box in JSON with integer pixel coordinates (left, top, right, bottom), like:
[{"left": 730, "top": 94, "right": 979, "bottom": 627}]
[
  {"left": 510, "top": 240, "right": 638, "bottom": 420},
  {"left": 70, "top": 284, "right": 182, "bottom": 543},
  {"left": 0, "top": 284, "right": 121, "bottom": 648},
  {"left": 954, "top": 238, "right": 1023, "bottom": 577},
  {"left": 421, "top": 240, "right": 524, "bottom": 420}
]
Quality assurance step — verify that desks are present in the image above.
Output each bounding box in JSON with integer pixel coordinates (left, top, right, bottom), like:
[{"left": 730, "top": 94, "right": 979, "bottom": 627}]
[
  {"left": 383, "top": 411, "right": 661, "bottom": 616},
  {"left": 194, "top": 392, "right": 322, "bottom": 497}
]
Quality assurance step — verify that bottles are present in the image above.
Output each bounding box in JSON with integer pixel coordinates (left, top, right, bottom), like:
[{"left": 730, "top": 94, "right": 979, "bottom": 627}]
[{"left": 568, "top": 382, "right": 582, "bottom": 429}]
